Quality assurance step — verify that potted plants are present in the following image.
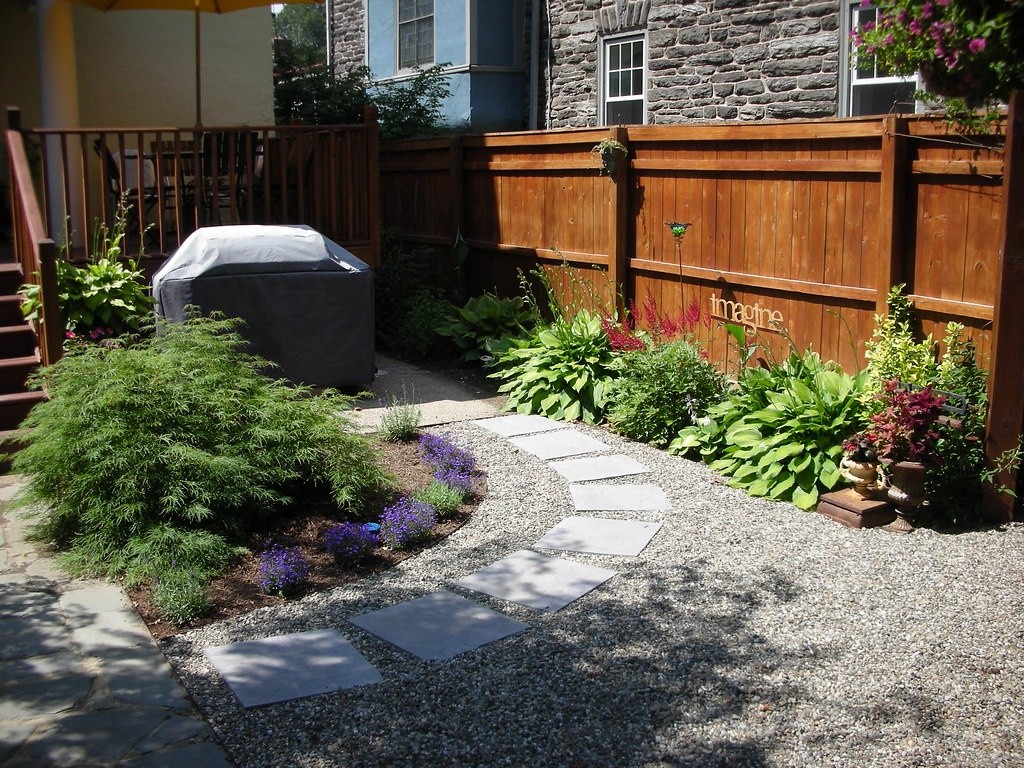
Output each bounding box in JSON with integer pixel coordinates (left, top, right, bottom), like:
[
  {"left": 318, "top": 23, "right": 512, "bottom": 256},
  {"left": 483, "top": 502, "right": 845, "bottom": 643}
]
[{"left": 591, "top": 138, "right": 628, "bottom": 180}]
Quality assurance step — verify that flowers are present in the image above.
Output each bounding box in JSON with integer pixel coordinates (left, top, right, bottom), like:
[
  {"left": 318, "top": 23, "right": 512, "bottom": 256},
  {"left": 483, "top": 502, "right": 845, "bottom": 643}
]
[
  {"left": 846, "top": 0, "right": 1024, "bottom": 75},
  {"left": 883, "top": 378, "right": 947, "bottom": 461},
  {"left": 840, "top": 427, "right": 881, "bottom": 461}
]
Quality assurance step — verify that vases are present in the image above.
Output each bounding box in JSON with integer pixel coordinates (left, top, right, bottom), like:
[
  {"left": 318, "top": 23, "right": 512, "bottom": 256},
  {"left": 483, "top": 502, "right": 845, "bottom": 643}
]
[
  {"left": 918, "top": 59, "right": 977, "bottom": 96},
  {"left": 882, "top": 463, "right": 925, "bottom": 535},
  {"left": 843, "top": 459, "right": 878, "bottom": 494}
]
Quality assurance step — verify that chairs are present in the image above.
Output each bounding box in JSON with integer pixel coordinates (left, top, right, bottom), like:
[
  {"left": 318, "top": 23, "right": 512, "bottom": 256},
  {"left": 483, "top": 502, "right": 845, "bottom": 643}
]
[
  {"left": 92, "top": 139, "right": 175, "bottom": 255},
  {"left": 151, "top": 139, "right": 203, "bottom": 209},
  {"left": 253, "top": 132, "right": 314, "bottom": 190},
  {"left": 203, "top": 131, "right": 259, "bottom": 225}
]
